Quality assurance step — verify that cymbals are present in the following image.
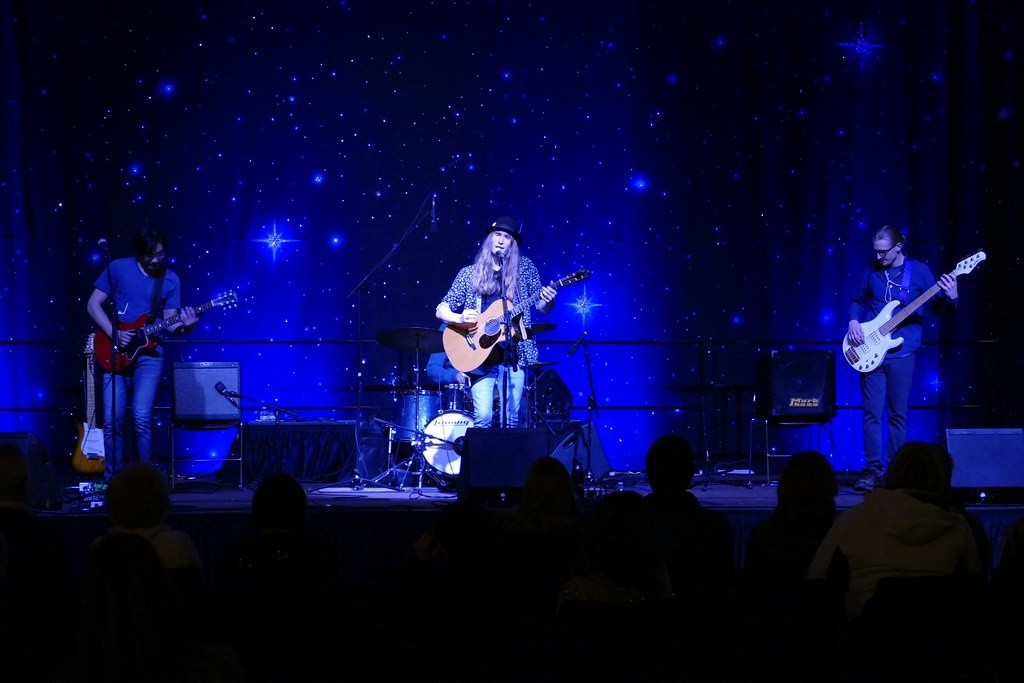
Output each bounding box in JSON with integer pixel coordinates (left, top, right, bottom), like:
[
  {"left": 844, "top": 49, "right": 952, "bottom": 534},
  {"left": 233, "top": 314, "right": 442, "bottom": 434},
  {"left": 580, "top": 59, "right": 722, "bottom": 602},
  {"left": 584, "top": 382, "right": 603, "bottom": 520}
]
[
  {"left": 532, "top": 324, "right": 558, "bottom": 333},
  {"left": 376, "top": 327, "right": 445, "bottom": 354},
  {"left": 522, "top": 360, "right": 556, "bottom": 367}
]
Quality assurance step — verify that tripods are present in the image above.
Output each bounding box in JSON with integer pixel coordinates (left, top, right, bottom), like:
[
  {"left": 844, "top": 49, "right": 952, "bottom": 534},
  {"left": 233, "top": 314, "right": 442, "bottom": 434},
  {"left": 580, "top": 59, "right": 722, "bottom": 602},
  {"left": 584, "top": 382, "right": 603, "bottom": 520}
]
[
  {"left": 306, "top": 210, "right": 449, "bottom": 495},
  {"left": 687, "top": 346, "right": 755, "bottom": 493}
]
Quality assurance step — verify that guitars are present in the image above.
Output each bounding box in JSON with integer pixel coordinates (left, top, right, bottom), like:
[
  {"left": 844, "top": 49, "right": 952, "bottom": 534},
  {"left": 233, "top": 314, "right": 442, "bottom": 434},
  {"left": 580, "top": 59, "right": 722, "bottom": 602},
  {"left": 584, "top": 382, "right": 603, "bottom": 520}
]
[
  {"left": 94, "top": 289, "right": 239, "bottom": 373},
  {"left": 443, "top": 269, "right": 592, "bottom": 378},
  {"left": 842, "top": 248, "right": 987, "bottom": 374},
  {"left": 71, "top": 331, "right": 106, "bottom": 474}
]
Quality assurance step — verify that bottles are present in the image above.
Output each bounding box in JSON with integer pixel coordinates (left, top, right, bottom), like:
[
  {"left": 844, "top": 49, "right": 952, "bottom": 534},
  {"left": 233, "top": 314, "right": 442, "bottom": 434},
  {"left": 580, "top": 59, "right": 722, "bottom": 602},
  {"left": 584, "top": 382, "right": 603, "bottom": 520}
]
[{"left": 616, "top": 482, "right": 624, "bottom": 492}]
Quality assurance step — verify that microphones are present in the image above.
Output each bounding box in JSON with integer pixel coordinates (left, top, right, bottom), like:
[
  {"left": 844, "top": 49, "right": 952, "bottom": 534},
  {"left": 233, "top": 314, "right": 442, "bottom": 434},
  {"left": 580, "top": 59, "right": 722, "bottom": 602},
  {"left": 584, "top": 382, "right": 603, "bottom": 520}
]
[
  {"left": 98, "top": 238, "right": 109, "bottom": 252},
  {"left": 569, "top": 334, "right": 586, "bottom": 354},
  {"left": 458, "top": 440, "right": 464, "bottom": 453},
  {"left": 214, "top": 381, "right": 236, "bottom": 406},
  {"left": 430, "top": 197, "right": 438, "bottom": 233}
]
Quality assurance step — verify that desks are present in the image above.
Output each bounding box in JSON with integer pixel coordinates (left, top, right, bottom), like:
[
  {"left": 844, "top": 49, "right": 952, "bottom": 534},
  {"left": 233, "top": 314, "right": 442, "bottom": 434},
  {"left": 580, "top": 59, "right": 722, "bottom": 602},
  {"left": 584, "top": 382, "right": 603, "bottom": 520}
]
[{"left": 221, "top": 420, "right": 364, "bottom": 488}]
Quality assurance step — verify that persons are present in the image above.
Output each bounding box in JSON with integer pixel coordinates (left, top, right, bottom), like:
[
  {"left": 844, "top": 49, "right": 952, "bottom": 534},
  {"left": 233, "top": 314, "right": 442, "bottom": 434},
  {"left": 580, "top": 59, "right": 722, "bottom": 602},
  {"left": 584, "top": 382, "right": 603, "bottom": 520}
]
[
  {"left": 398, "top": 501, "right": 500, "bottom": 623},
  {"left": 435, "top": 215, "right": 558, "bottom": 428},
  {"left": 43, "top": 533, "right": 250, "bottom": 683},
  {"left": 749, "top": 454, "right": 836, "bottom": 581},
  {"left": 500, "top": 456, "right": 586, "bottom": 543},
  {"left": 847, "top": 223, "right": 959, "bottom": 489},
  {"left": 552, "top": 489, "right": 674, "bottom": 624},
  {"left": 804, "top": 443, "right": 984, "bottom": 577},
  {"left": 645, "top": 435, "right": 733, "bottom": 610},
  {"left": 0, "top": 446, "right": 77, "bottom": 653},
  {"left": 89, "top": 461, "right": 205, "bottom": 589},
  {"left": 206, "top": 476, "right": 340, "bottom": 631},
  {"left": 979, "top": 514, "right": 1024, "bottom": 682},
  {"left": 927, "top": 445, "right": 993, "bottom": 586},
  {"left": 87, "top": 222, "right": 198, "bottom": 484}
]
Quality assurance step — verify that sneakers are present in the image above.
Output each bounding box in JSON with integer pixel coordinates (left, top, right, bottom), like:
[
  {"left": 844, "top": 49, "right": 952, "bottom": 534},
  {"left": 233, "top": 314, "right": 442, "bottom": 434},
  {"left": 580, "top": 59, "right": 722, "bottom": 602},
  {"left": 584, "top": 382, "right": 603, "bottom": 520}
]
[{"left": 854, "top": 470, "right": 880, "bottom": 490}]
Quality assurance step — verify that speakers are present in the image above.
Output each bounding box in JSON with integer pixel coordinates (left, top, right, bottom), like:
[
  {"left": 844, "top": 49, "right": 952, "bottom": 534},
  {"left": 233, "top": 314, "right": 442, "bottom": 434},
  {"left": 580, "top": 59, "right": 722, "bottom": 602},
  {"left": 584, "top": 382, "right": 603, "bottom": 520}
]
[
  {"left": 527, "top": 368, "right": 579, "bottom": 430},
  {"left": 172, "top": 361, "right": 243, "bottom": 423},
  {"left": 457, "top": 427, "right": 548, "bottom": 505},
  {"left": 531, "top": 419, "right": 610, "bottom": 484},
  {"left": 771, "top": 349, "right": 837, "bottom": 422},
  {"left": 940, "top": 428, "right": 1024, "bottom": 506},
  {"left": 0, "top": 429, "right": 65, "bottom": 512}
]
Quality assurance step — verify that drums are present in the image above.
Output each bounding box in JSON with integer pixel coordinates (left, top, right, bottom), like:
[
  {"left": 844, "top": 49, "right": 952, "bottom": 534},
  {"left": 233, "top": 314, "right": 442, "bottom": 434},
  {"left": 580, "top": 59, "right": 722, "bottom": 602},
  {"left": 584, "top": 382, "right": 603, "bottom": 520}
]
[
  {"left": 440, "top": 382, "right": 475, "bottom": 414},
  {"left": 393, "top": 385, "right": 444, "bottom": 443},
  {"left": 419, "top": 408, "right": 474, "bottom": 477}
]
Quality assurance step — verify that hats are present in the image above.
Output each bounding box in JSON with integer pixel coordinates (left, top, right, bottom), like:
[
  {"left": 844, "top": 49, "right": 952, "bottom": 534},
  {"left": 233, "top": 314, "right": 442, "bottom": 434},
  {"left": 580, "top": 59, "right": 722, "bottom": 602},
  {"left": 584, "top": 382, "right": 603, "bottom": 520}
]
[{"left": 486, "top": 217, "right": 522, "bottom": 245}]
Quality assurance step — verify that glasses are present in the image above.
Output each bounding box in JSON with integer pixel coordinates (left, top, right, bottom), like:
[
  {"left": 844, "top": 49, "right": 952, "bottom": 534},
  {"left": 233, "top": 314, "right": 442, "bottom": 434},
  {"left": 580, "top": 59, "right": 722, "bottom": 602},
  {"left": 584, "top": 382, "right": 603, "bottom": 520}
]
[{"left": 872, "top": 241, "right": 900, "bottom": 257}]
